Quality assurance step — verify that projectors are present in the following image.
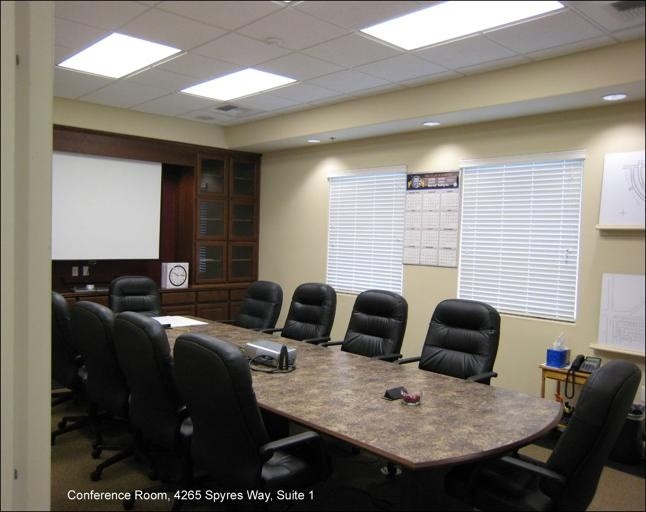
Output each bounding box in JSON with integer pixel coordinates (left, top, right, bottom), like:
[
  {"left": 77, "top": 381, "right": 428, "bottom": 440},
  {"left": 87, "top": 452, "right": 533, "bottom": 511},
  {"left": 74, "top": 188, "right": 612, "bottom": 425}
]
[{"left": 245, "top": 341, "right": 296, "bottom": 367}]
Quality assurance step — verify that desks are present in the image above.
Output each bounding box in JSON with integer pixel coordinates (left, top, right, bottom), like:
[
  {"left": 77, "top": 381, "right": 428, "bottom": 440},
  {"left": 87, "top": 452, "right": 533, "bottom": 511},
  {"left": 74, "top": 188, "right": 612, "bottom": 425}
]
[
  {"left": 153, "top": 313, "right": 564, "bottom": 470},
  {"left": 539, "top": 362, "right": 592, "bottom": 399}
]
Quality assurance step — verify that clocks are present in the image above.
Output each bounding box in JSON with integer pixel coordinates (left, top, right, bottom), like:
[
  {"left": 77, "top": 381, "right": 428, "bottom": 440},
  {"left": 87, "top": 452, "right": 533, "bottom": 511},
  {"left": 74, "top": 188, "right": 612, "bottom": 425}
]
[{"left": 161, "top": 262, "right": 189, "bottom": 290}]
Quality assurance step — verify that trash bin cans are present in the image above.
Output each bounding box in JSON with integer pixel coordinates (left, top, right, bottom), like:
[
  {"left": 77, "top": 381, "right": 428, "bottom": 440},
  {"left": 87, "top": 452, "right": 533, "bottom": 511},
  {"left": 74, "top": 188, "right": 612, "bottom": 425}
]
[{"left": 610, "top": 405, "right": 645, "bottom": 465}]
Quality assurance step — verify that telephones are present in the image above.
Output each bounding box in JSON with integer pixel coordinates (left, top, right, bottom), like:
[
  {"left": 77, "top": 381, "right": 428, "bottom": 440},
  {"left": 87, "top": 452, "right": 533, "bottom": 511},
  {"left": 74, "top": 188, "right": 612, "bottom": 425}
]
[{"left": 571, "top": 355, "right": 601, "bottom": 374}]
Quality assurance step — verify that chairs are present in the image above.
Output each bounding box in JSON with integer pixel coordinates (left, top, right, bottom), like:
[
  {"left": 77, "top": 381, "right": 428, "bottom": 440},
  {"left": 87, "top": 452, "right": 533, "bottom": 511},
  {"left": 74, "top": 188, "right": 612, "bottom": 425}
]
[
  {"left": 442, "top": 358, "right": 642, "bottom": 511},
  {"left": 109, "top": 275, "right": 162, "bottom": 315},
  {"left": 220, "top": 277, "right": 501, "bottom": 385},
  {"left": 50, "top": 291, "right": 321, "bottom": 512}
]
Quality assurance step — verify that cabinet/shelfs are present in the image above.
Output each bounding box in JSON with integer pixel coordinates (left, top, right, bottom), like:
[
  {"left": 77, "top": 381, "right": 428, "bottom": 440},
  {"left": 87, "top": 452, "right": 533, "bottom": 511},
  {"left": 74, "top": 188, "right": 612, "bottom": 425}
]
[{"left": 172, "top": 151, "right": 261, "bottom": 286}]
